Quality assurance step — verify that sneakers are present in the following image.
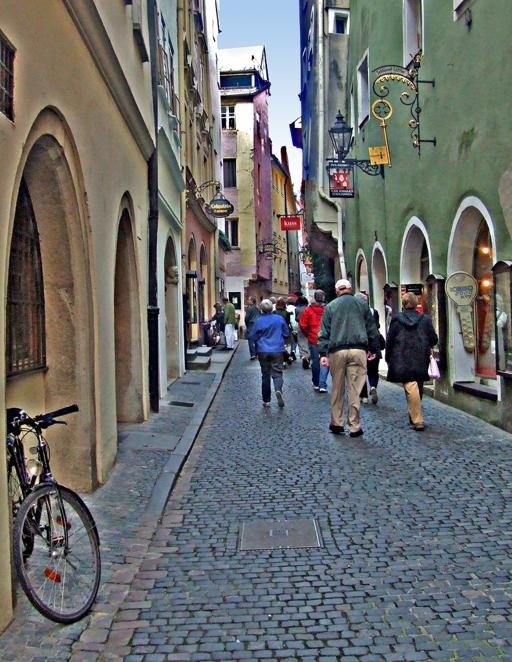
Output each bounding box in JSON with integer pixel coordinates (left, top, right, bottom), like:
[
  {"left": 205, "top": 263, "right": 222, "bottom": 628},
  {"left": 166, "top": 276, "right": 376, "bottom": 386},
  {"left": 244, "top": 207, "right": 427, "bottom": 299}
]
[
  {"left": 276, "top": 391, "right": 284, "bottom": 407},
  {"left": 308, "top": 366, "right": 309, "bottom": 366},
  {"left": 302, "top": 356, "right": 310, "bottom": 369},
  {"left": 262, "top": 401, "right": 270, "bottom": 406},
  {"left": 371, "top": 386, "right": 378, "bottom": 404},
  {"left": 361, "top": 397, "right": 368, "bottom": 404}
]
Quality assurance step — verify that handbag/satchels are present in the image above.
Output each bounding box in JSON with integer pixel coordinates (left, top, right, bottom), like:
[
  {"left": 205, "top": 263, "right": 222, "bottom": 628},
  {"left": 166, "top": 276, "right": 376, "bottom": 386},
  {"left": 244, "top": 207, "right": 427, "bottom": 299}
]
[
  {"left": 428, "top": 350, "right": 440, "bottom": 379},
  {"left": 377, "top": 331, "right": 386, "bottom": 350}
]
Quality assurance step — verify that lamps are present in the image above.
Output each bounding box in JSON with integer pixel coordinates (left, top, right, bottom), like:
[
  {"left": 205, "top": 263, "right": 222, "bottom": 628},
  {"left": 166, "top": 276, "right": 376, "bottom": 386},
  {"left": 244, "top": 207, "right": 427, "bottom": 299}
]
[{"left": 327, "top": 109, "right": 385, "bottom": 182}]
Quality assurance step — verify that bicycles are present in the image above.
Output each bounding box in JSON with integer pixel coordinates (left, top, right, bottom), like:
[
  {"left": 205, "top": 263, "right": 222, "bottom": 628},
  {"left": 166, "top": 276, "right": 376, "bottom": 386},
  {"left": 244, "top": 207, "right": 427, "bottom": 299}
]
[{"left": 8, "top": 400, "right": 106, "bottom": 626}]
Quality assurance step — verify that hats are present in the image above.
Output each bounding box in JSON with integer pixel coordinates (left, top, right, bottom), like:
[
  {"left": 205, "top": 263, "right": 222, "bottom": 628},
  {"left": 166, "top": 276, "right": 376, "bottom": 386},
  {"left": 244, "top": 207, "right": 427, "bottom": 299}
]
[{"left": 335, "top": 279, "right": 351, "bottom": 290}]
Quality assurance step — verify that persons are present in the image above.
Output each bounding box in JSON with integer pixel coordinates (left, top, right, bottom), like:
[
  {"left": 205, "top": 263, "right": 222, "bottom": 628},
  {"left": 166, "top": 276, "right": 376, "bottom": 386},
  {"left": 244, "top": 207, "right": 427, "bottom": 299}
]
[
  {"left": 207, "top": 289, "right": 386, "bottom": 406},
  {"left": 342, "top": 168, "right": 351, "bottom": 190},
  {"left": 384, "top": 291, "right": 440, "bottom": 431},
  {"left": 316, "top": 279, "right": 379, "bottom": 437},
  {"left": 332, "top": 169, "right": 341, "bottom": 190},
  {"left": 245, "top": 299, "right": 290, "bottom": 408}
]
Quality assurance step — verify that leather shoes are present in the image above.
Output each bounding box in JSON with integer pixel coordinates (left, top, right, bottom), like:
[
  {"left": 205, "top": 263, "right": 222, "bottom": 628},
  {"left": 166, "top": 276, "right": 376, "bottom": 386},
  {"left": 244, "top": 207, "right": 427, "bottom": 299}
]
[
  {"left": 314, "top": 385, "right": 319, "bottom": 391},
  {"left": 330, "top": 423, "right": 344, "bottom": 432},
  {"left": 350, "top": 429, "right": 363, "bottom": 437},
  {"left": 319, "top": 388, "right": 328, "bottom": 393},
  {"left": 413, "top": 422, "right": 425, "bottom": 429}
]
